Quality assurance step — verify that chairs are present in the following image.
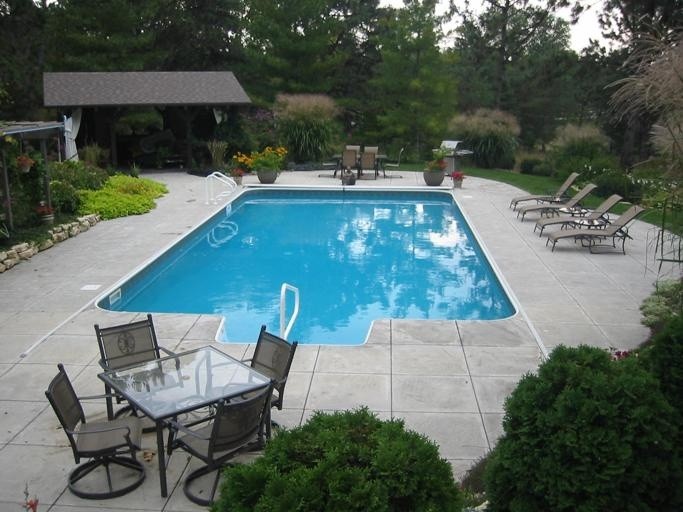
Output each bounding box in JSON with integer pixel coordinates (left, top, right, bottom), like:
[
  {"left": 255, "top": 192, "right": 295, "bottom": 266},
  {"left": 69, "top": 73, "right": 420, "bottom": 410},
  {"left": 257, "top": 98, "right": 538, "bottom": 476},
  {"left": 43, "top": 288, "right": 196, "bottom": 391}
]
[
  {"left": 45, "top": 363, "right": 146, "bottom": 500},
  {"left": 534, "top": 194, "right": 623, "bottom": 237},
  {"left": 167, "top": 378, "right": 276, "bottom": 506},
  {"left": 545, "top": 203, "right": 645, "bottom": 253},
  {"left": 510, "top": 172, "right": 580, "bottom": 212},
  {"left": 319, "top": 145, "right": 404, "bottom": 180},
  {"left": 92, "top": 313, "right": 179, "bottom": 433},
  {"left": 517, "top": 182, "right": 597, "bottom": 222},
  {"left": 210, "top": 324, "right": 298, "bottom": 428}
]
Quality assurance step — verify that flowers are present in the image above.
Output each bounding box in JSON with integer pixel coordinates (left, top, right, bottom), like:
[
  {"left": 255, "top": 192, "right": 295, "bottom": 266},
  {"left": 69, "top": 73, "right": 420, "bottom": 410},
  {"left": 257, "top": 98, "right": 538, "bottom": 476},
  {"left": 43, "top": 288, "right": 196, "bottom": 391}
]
[
  {"left": 229, "top": 169, "right": 245, "bottom": 176},
  {"left": 451, "top": 171, "right": 463, "bottom": 180},
  {"left": 232, "top": 144, "right": 289, "bottom": 168},
  {"left": 35, "top": 206, "right": 53, "bottom": 215}
]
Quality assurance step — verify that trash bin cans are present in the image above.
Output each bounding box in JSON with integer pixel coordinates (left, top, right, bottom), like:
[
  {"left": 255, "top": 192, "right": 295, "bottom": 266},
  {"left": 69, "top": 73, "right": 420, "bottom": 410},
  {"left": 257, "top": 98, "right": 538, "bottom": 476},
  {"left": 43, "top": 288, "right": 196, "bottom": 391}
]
[{"left": 432, "top": 140, "right": 474, "bottom": 177}]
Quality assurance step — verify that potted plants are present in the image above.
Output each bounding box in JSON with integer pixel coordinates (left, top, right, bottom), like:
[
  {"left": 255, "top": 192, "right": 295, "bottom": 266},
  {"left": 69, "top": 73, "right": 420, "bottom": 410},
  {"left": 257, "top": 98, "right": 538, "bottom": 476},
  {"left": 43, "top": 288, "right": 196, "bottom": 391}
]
[{"left": 422, "top": 147, "right": 448, "bottom": 186}]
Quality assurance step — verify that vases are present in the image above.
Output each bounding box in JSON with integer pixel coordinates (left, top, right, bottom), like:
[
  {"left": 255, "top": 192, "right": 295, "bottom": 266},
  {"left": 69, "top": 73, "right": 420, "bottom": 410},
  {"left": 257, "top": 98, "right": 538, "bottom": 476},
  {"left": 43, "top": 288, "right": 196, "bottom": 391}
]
[
  {"left": 234, "top": 176, "right": 242, "bottom": 185},
  {"left": 258, "top": 168, "right": 278, "bottom": 183},
  {"left": 453, "top": 178, "right": 463, "bottom": 189},
  {"left": 41, "top": 215, "right": 55, "bottom": 226}
]
